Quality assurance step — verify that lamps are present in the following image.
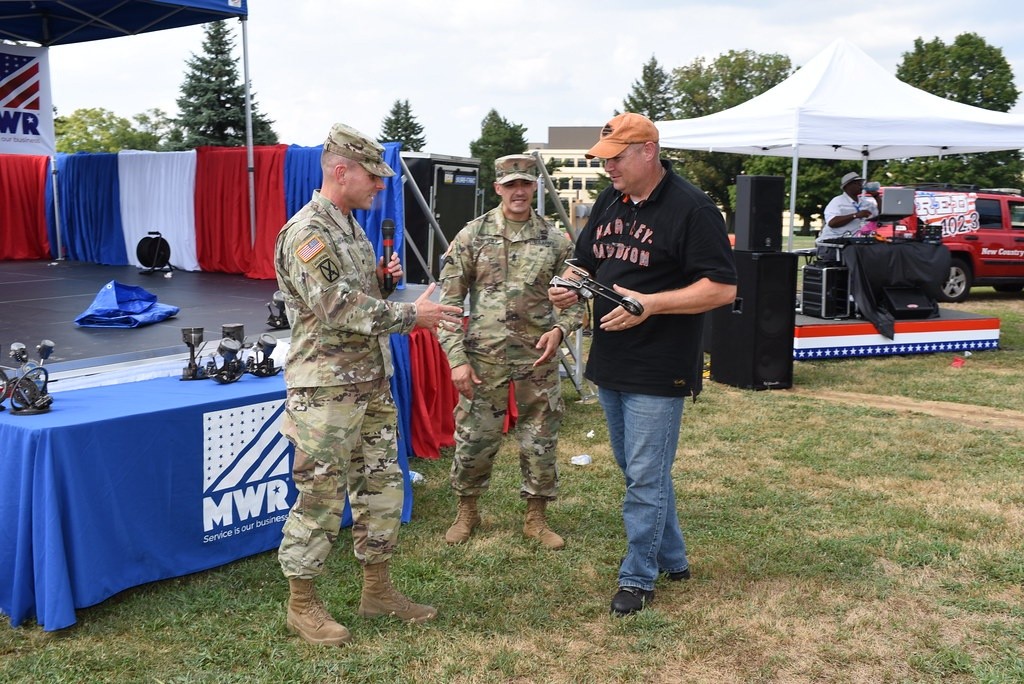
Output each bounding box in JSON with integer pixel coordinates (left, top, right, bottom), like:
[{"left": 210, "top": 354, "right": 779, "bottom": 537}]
[
  {"left": 0, "top": 339, "right": 56, "bottom": 415},
  {"left": 180, "top": 290, "right": 290, "bottom": 384}
]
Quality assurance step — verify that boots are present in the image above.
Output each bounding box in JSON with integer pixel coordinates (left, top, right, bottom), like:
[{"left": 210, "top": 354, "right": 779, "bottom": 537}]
[
  {"left": 522, "top": 498, "right": 565, "bottom": 551},
  {"left": 444, "top": 495, "right": 482, "bottom": 546},
  {"left": 357, "top": 558, "right": 439, "bottom": 624},
  {"left": 287, "top": 576, "right": 350, "bottom": 645}
]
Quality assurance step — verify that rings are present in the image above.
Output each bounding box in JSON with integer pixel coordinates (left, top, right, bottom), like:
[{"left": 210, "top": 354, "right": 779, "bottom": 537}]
[
  {"left": 622, "top": 321, "right": 627, "bottom": 327},
  {"left": 438, "top": 321, "right": 444, "bottom": 330}
]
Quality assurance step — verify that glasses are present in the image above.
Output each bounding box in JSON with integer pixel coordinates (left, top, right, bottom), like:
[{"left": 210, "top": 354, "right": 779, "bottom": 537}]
[{"left": 852, "top": 180, "right": 863, "bottom": 184}]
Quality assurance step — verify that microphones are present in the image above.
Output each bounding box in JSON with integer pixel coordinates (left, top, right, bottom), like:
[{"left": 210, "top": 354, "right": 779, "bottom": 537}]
[{"left": 380, "top": 218, "right": 396, "bottom": 292}]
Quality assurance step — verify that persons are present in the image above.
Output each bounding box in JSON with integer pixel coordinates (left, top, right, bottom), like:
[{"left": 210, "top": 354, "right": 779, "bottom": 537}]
[
  {"left": 274, "top": 123, "right": 463, "bottom": 647},
  {"left": 548, "top": 112, "right": 739, "bottom": 619},
  {"left": 816, "top": 173, "right": 882, "bottom": 261},
  {"left": 436, "top": 154, "right": 586, "bottom": 550}
]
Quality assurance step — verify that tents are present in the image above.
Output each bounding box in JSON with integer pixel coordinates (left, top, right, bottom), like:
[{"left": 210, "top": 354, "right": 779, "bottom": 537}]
[
  {"left": 0, "top": 0, "right": 256, "bottom": 259},
  {"left": 654, "top": 34, "right": 1024, "bottom": 254}
]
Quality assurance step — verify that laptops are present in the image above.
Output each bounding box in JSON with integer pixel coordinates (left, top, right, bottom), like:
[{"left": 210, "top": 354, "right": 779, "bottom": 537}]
[{"left": 866, "top": 188, "right": 915, "bottom": 221}]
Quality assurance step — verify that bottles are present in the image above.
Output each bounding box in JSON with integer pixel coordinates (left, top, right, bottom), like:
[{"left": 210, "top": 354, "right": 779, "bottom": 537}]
[
  {"left": 408, "top": 470, "right": 427, "bottom": 483},
  {"left": 571, "top": 456, "right": 592, "bottom": 465}
]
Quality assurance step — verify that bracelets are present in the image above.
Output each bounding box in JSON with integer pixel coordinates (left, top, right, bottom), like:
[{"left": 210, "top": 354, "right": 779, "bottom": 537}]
[
  {"left": 853, "top": 213, "right": 857, "bottom": 218},
  {"left": 873, "top": 193, "right": 880, "bottom": 198}
]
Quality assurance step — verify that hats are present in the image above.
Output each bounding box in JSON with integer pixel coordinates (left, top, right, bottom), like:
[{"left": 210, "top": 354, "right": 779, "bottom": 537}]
[
  {"left": 840, "top": 172, "right": 866, "bottom": 189},
  {"left": 323, "top": 124, "right": 397, "bottom": 177},
  {"left": 495, "top": 154, "right": 537, "bottom": 184},
  {"left": 585, "top": 112, "right": 659, "bottom": 159}
]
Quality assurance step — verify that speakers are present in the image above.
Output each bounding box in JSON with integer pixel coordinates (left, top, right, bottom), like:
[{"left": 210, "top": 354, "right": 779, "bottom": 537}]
[
  {"left": 883, "top": 286, "right": 933, "bottom": 320},
  {"left": 710, "top": 249, "right": 798, "bottom": 391},
  {"left": 734, "top": 176, "right": 785, "bottom": 253}
]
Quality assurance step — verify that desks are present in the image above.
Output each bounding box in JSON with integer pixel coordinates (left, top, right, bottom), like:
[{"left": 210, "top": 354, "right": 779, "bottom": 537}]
[{"left": 0, "top": 365, "right": 351, "bottom": 630}]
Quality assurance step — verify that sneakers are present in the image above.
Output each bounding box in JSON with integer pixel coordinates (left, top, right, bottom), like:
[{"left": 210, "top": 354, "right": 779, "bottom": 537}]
[
  {"left": 609, "top": 587, "right": 654, "bottom": 617},
  {"left": 620, "top": 555, "right": 691, "bottom": 582}
]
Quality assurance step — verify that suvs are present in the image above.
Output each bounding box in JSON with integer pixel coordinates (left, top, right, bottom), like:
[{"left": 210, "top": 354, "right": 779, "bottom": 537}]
[{"left": 857, "top": 183, "right": 1024, "bottom": 303}]
